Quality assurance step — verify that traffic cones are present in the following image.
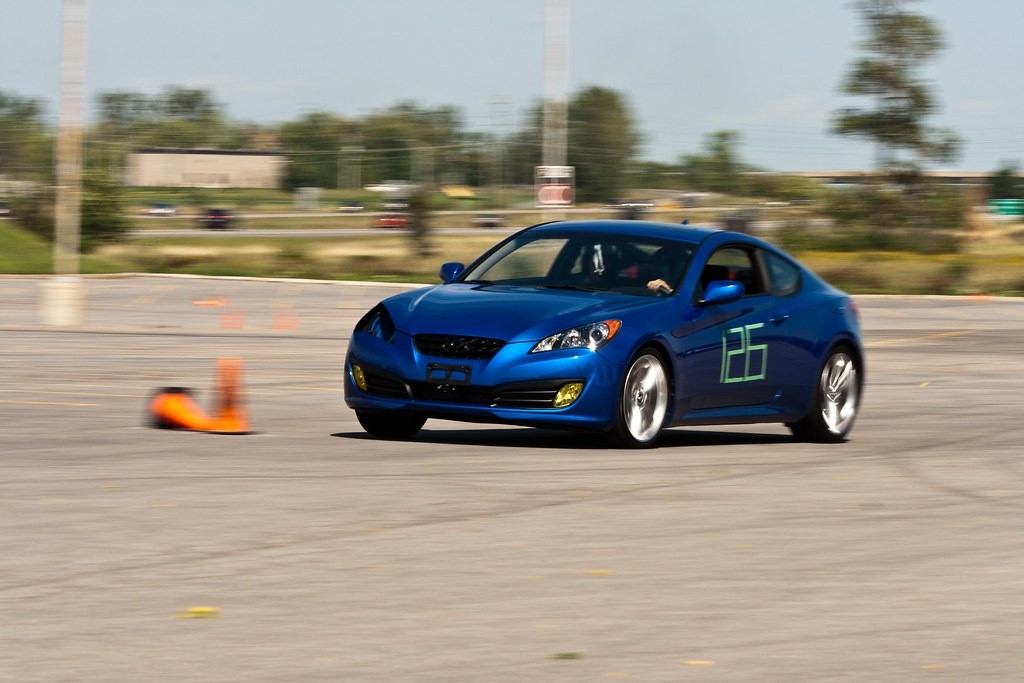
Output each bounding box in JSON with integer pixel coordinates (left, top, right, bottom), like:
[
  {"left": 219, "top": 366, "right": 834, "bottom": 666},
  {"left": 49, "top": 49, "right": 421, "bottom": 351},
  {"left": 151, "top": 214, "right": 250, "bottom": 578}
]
[
  {"left": 204, "top": 355, "right": 255, "bottom": 437},
  {"left": 145, "top": 385, "right": 217, "bottom": 431}
]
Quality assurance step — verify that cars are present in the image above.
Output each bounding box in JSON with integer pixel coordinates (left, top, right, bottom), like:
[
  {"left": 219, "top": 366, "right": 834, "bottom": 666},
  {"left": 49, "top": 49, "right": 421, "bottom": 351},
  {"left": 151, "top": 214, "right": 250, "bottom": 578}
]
[{"left": 343, "top": 220, "right": 865, "bottom": 449}]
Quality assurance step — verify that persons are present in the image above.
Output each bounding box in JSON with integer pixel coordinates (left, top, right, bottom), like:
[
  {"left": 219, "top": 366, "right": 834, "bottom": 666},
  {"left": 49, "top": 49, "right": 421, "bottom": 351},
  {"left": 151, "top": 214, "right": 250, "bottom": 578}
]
[{"left": 629, "top": 246, "right": 691, "bottom": 294}]
